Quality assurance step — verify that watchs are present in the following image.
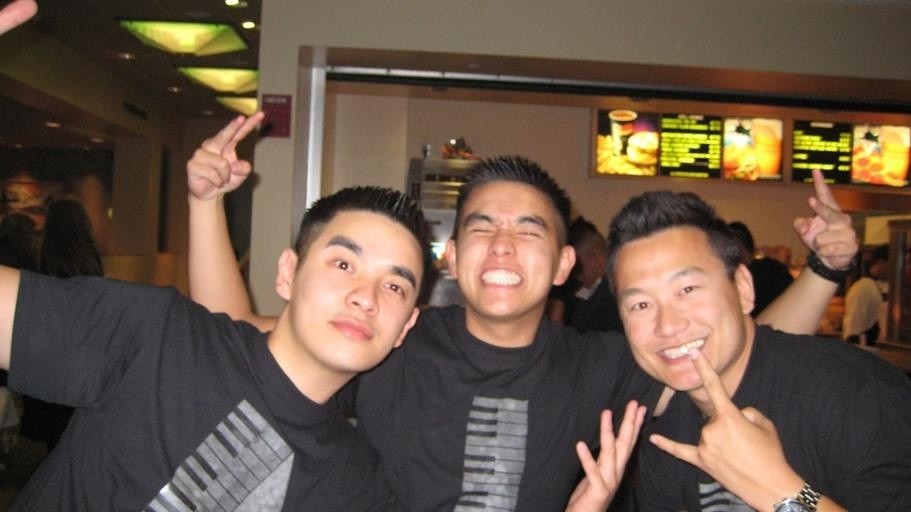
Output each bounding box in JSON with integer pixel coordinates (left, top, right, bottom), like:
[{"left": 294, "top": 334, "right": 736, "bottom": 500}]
[{"left": 774, "top": 483, "right": 821, "bottom": 512}]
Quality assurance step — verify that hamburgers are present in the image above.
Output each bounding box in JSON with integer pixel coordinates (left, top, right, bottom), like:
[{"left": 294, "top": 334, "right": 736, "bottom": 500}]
[{"left": 625, "top": 130, "right": 659, "bottom": 166}]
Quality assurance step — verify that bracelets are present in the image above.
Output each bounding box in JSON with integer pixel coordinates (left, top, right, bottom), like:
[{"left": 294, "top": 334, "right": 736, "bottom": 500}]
[{"left": 807, "top": 249, "right": 845, "bottom": 284}]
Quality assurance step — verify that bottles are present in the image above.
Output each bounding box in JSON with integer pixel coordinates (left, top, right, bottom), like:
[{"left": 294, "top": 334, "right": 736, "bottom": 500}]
[{"left": 423, "top": 144, "right": 432, "bottom": 159}]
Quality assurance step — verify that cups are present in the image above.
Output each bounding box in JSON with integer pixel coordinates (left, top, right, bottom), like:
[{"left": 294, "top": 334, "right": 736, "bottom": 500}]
[
  {"left": 609, "top": 110, "right": 638, "bottom": 157},
  {"left": 750, "top": 118, "right": 782, "bottom": 180},
  {"left": 877, "top": 127, "right": 911, "bottom": 182}
]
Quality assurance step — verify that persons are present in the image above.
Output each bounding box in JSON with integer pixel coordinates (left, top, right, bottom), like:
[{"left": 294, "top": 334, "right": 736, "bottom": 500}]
[
  {"left": 0, "top": 212, "right": 36, "bottom": 269},
  {"left": 842, "top": 243, "right": 889, "bottom": 347},
  {"left": 604, "top": 190, "right": 911, "bottom": 512},
  {"left": 19, "top": 194, "right": 104, "bottom": 453},
  {"left": 0, "top": 0, "right": 647, "bottom": 512},
  {"left": 728, "top": 222, "right": 795, "bottom": 319},
  {"left": 186, "top": 111, "right": 859, "bottom": 512},
  {"left": 548, "top": 214, "right": 625, "bottom": 334}
]
